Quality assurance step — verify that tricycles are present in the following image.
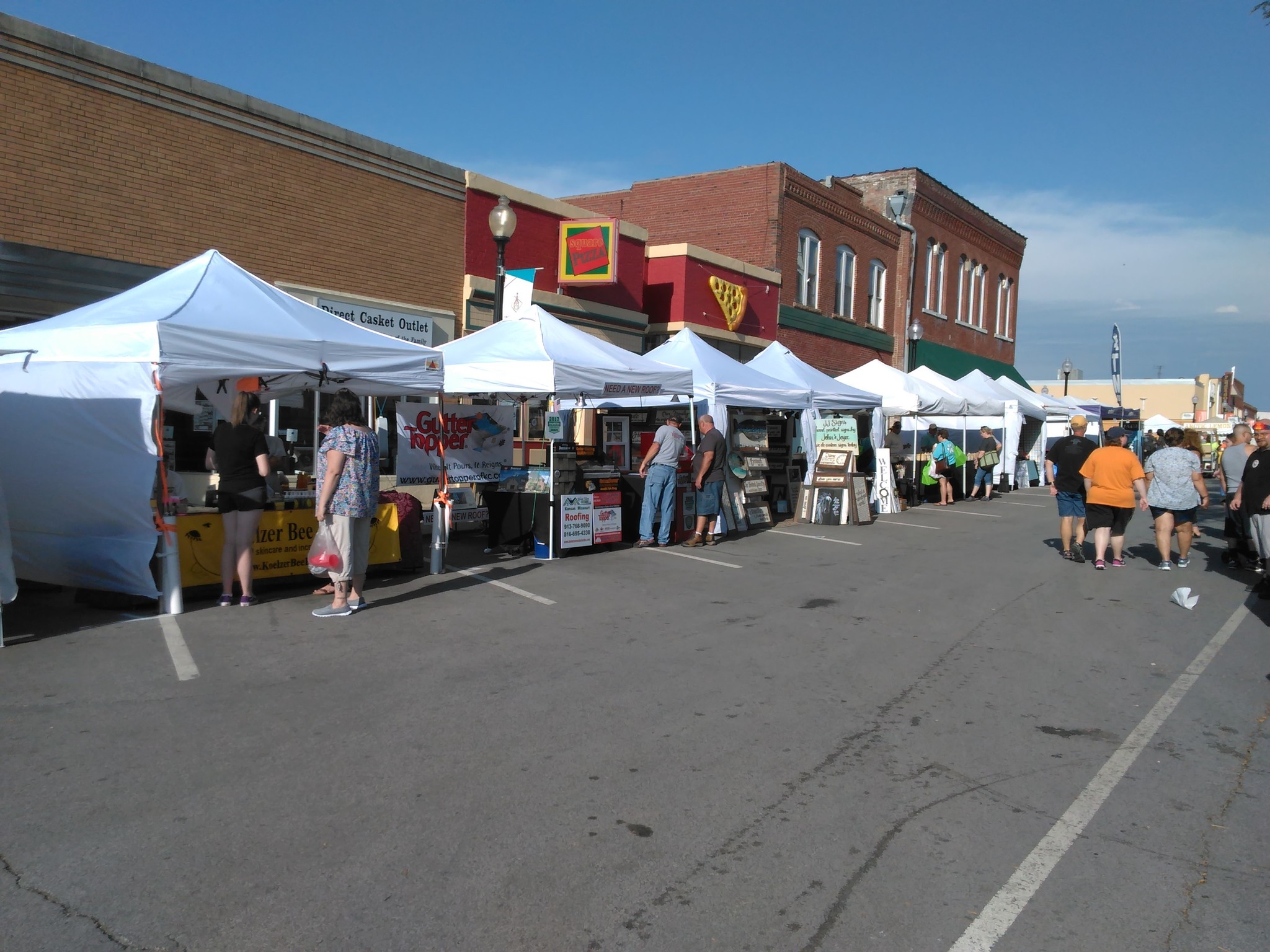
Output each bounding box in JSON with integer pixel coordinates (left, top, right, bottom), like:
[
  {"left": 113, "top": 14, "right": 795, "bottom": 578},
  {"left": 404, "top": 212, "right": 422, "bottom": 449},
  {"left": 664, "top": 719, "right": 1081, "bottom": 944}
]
[{"left": 1183, "top": 426, "right": 1219, "bottom": 479}]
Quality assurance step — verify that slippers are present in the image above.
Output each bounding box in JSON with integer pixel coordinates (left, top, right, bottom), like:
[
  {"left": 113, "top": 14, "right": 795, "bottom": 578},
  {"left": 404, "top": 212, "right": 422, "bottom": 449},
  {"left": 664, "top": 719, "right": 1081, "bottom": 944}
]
[
  {"left": 934, "top": 502, "right": 947, "bottom": 506},
  {"left": 947, "top": 502, "right": 956, "bottom": 504}
]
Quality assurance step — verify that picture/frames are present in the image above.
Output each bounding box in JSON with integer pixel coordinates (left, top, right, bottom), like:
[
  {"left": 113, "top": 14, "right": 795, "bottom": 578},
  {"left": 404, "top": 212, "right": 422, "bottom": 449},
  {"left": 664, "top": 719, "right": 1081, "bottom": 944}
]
[
  {"left": 847, "top": 471, "right": 873, "bottom": 527},
  {"left": 729, "top": 405, "right": 801, "bottom": 529},
  {"left": 810, "top": 471, "right": 849, "bottom": 490},
  {"left": 796, "top": 483, "right": 815, "bottom": 525},
  {"left": 814, "top": 449, "right": 853, "bottom": 470}
]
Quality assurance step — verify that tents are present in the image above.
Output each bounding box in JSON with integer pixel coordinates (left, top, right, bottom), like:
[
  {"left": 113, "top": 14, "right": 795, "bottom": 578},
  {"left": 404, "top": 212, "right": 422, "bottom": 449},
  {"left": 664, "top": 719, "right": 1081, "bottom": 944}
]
[
  {"left": 745, "top": 340, "right": 1270, "bottom": 538},
  {"left": 428, "top": 302, "right": 698, "bottom": 557},
  {"left": 553, "top": 326, "right": 819, "bottom": 532},
  {"left": 0, "top": 245, "right": 449, "bottom": 613}
]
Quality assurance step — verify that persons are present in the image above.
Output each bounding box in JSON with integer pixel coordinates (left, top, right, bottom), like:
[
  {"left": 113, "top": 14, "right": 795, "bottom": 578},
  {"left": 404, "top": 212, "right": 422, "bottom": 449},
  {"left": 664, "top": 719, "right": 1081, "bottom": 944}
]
[
  {"left": 1078, "top": 426, "right": 1148, "bottom": 571},
  {"left": 633, "top": 413, "right": 686, "bottom": 547},
  {"left": 1219, "top": 424, "right": 1262, "bottom": 570},
  {"left": 313, "top": 577, "right": 367, "bottom": 596},
  {"left": 681, "top": 414, "right": 727, "bottom": 548},
  {"left": 1144, "top": 427, "right": 1210, "bottom": 571},
  {"left": 205, "top": 390, "right": 270, "bottom": 608},
  {"left": 1044, "top": 414, "right": 1099, "bottom": 563},
  {"left": 155, "top": 449, "right": 191, "bottom": 505},
  {"left": 312, "top": 391, "right": 381, "bottom": 617},
  {"left": 249, "top": 409, "right": 288, "bottom": 494},
  {"left": 1229, "top": 418, "right": 1270, "bottom": 599}
]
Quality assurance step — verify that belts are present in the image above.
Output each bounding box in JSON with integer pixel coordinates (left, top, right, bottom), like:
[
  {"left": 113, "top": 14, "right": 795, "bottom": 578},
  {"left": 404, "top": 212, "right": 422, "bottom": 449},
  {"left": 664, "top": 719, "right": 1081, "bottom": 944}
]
[{"left": 652, "top": 462, "right": 677, "bottom": 470}]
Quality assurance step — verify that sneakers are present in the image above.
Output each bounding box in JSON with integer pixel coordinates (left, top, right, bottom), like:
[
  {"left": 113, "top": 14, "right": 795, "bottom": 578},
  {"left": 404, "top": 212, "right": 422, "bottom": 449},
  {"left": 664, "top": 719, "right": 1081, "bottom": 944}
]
[
  {"left": 240, "top": 595, "right": 258, "bottom": 606},
  {"left": 1158, "top": 560, "right": 1171, "bottom": 571},
  {"left": 219, "top": 594, "right": 233, "bottom": 606},
  {"left": 1177, "top": 557, "right": 1190, "bottom": 567},
  {"left": 1112, "top": 558, "right": 1126, "bottom": 567},
  {"left": 1095, "top": 560, "right": 1107, "bottom": 569}
]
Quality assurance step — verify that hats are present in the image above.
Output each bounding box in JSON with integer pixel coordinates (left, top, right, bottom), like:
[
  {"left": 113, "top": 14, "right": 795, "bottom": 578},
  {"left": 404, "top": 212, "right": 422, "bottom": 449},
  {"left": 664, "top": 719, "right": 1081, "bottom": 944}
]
[
  {"left": 892, "top": 421, "right": 901, "bottom": 428},
  {"left": 929, "top": 424, "right": 939, "bottom": 429},
  {"left": 1070, "top": 415, "right": 1089, "bottom": 427},
  {"left": 1106, "top": 426, "right": 1133, "bottom": 440},
  {"left": 669, "top": 413, "right": 682, "bottom": 426}
]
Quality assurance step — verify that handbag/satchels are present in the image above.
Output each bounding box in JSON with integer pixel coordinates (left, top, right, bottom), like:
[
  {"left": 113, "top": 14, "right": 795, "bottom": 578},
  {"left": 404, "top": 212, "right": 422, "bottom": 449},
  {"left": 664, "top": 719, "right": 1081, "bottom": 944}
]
[
  {"left": 307, "top": 519, "right": 341, "bottom": 575},
  {"left": 977, "top": 450, "right": 999, "bottom": 470},
  {"left": 921, "top": 442, "right": 950, "bottom": 485},
  {"left": 954, "top": 443, "right": 968, "bottom": 468}
]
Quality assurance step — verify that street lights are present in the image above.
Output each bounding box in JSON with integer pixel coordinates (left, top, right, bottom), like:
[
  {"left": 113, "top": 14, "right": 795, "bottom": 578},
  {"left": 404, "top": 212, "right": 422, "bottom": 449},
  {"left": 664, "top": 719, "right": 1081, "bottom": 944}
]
[
  {"left": 486, "top": 193, "right": 517, "bottom": 324},
  {"left": 1061, "top": 356, "right": 1073, "bottom": 396},
  {"left": 911, "top": 317, "right": 924, "bottom": 374}
]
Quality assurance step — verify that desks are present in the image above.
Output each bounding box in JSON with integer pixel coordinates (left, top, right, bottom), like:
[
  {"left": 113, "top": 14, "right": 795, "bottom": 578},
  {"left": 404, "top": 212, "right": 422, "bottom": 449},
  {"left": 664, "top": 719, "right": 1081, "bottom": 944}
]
[{"left": 163, "top": 494, "right": 401, "bottom": 590}]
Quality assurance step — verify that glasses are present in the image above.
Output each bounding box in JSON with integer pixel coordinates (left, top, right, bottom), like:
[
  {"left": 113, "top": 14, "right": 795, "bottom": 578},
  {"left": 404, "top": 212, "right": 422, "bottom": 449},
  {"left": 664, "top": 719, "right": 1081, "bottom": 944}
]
[{"left": 1245, "top": 431, "right": 1254, "bottom": 436}]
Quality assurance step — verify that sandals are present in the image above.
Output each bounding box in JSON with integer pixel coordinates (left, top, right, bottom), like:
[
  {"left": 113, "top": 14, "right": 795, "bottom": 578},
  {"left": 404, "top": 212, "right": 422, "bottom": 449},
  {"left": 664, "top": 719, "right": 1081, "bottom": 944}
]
[
  {"left": 1063, "top": 543, "right": 1085, "bottom": 562},
  {"left": 313, "top": 582, "right": 335, "bottom": 596}
]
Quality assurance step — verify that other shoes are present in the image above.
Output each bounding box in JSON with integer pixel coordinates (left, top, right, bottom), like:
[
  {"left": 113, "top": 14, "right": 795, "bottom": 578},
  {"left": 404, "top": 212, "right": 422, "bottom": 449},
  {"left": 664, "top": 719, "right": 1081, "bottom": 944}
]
[
  {"left": 312, "top": 603, "right": 352, "bottom": 617},
  {"left": 633, "top": 539, "right": 658, "bottom": 547},
  {"left": 681, "top": 531, "right": 703, "bottom": 547},
  {"left": 703, "top": 532, "right": 716, "bottom": 545},
  {"left": 965, "top": 497, "right": 975, "bottom": 501},
  {"left": 1221, "top": 549, "right": 1270, "bottom": 600},
  {"left": 659, "top": 543, "right": 668, "bottom": 547},
  {"left": 347, "top": 597, "right": 368, "bottom": 609},
  {"left": 980, "top": 496, "right": 989, "bottom": 501}
]
